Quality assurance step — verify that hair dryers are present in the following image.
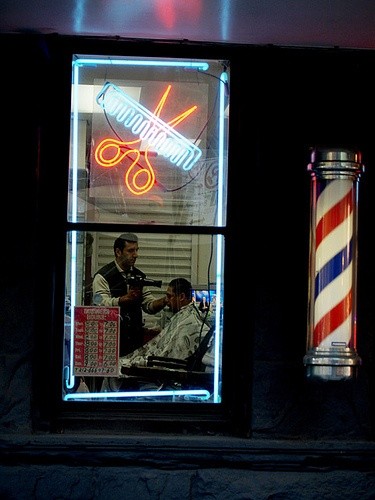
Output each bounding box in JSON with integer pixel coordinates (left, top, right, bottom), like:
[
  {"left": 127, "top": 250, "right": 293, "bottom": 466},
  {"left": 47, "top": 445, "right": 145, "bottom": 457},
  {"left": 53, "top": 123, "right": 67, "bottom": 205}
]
[{"left": 124, "top": 273, "right": 164, "bottom": 294}]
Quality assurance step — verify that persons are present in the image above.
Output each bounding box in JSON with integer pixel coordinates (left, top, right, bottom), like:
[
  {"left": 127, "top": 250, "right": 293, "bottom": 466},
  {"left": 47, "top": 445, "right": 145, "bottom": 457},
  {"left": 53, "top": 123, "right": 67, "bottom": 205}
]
[
  {"left": 200, "top": 297, "right": 209, "bottom": 312},
  {"left": 121, "top": 278, "right": 215, "bottom": 371},
  {"left": 91, "top": 232, "right": 168, "bottom": 358}
]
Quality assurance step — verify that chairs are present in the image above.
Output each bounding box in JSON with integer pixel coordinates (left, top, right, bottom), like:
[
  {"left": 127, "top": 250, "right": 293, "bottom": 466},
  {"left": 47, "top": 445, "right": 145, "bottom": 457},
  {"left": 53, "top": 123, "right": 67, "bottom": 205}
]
[{"left": 109, "top": 324, "right": 218, "bottom": 393}]
